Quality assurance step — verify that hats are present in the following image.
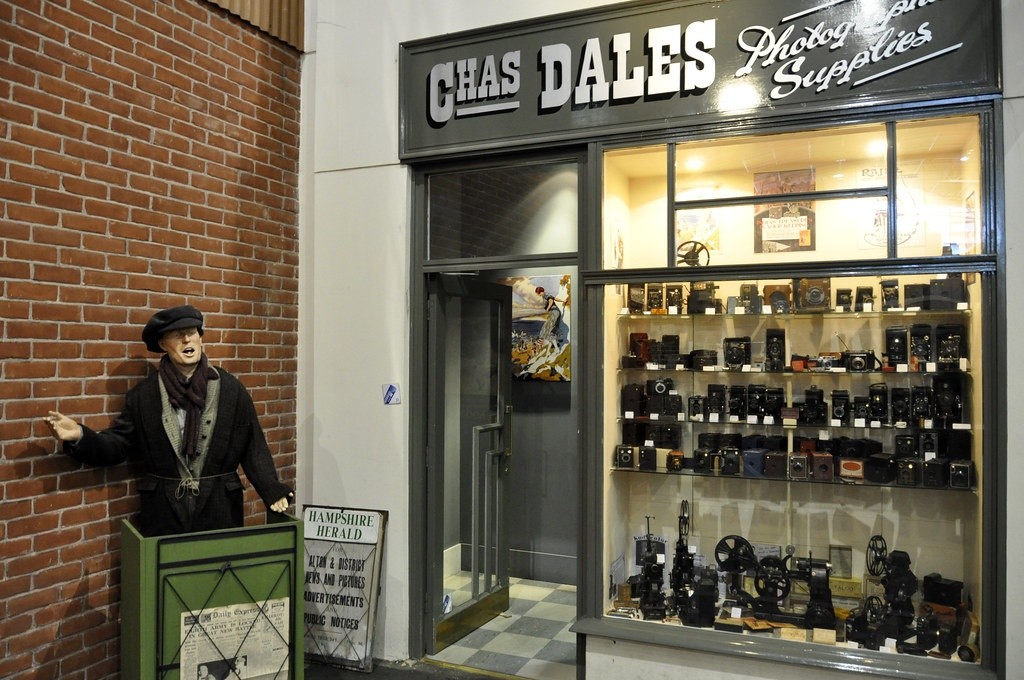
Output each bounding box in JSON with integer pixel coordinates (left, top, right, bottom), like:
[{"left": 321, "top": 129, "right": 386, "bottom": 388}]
[{"left": 142, "top": 306, "right": 204, "bottom": 353}]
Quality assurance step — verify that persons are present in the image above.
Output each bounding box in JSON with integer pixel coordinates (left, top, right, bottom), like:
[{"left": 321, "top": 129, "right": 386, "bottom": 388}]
[{"left": 42, "top": 305, "right": 297, "bottom": 537}]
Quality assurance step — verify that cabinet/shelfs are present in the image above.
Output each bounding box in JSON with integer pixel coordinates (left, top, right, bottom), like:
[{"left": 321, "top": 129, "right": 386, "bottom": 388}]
[{"left": 610, "top": 310, "right": 977, "bottom": 495}]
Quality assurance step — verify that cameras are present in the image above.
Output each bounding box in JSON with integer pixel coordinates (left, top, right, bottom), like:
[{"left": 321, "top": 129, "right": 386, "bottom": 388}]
[{"left": 617, "top": 277, "right": 977, "bottom": 489}]
